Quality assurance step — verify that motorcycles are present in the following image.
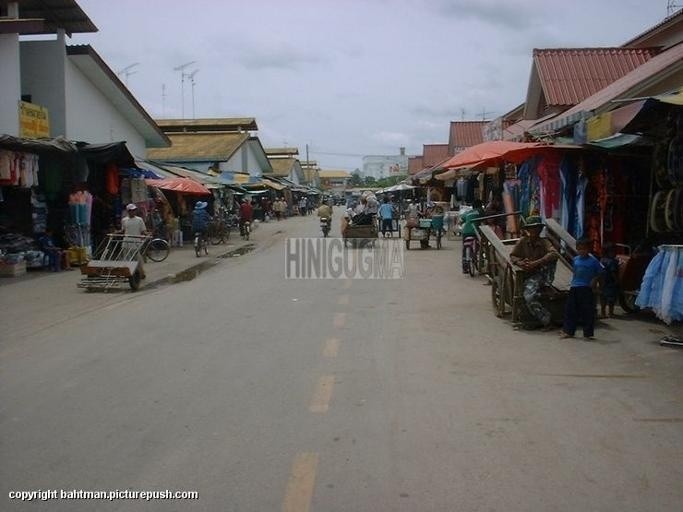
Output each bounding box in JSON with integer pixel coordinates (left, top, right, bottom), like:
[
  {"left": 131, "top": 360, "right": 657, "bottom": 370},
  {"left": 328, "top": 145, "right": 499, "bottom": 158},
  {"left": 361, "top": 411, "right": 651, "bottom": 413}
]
[{"left": 317, "top": 211, "right": 334, "bottom": 237}]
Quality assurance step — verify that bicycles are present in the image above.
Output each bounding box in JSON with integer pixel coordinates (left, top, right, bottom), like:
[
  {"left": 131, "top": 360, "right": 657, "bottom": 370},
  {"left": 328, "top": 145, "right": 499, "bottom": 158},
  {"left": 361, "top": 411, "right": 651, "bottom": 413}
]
[
  {"left": 462, "top": 231, "right": 486, "bottom": 276},
  {"left": 194, "top": 218, "right": 214, "bottom": 257},
  {"left": 144, "top": 238, "right": 171, "bottom": 263},
  {"left": 239, "top": 215, "right": 252, "bottom": 241},
  {"left": 209, "top": 211, "right": 231, "bottom": 244}
]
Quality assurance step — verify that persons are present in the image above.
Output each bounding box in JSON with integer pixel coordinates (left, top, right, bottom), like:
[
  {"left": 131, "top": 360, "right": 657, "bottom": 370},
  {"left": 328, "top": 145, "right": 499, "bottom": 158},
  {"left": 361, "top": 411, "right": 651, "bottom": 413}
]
[
  {"left": 599, "top": 239, "right": 620, "bottom": 319},
  {"left": 631, "top": 233, "right": 678, "bottom": 291},
  {"left": 554, "top": 236, "right": 603, "bottom": 342},
  {"left": 507, "top": 215, "right": 560, "bottom": 332},
  {"left": 41, "top": 227, "right": 77, "bottom": 272},
  {"left": 111, "top": 202, "right": 153, "bottom": 284},
  {"left": 151, "top": 196, "right": 507, "bottom": 287}
]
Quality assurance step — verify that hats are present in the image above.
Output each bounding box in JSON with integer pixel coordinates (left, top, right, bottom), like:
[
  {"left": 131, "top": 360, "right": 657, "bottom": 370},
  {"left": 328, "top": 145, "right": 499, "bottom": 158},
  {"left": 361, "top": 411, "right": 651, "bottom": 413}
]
[
  {"left": 521, "top": 215, "right": 546, "bottom": 230},
  {"left": 194, "top": 201, "right": 208, "bottom": 210},
  {"left": 242, "top": 197, "right": 249, "bottom": 203},
  {"left": 126, "top": 203, "right": 138, "bottom": 212}
]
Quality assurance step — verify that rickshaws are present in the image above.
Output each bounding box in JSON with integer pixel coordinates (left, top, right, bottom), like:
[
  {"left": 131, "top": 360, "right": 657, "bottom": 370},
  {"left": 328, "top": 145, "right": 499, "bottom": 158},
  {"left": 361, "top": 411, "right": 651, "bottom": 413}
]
[
  {"left": 404, "top": 208, "right": 442, "bottom": 251},
  {"left": 341, "top": 213, "right": 378, "bottom": 249},
  {"left": 467, "top": 210, "right": 606, "bottom": 332}
]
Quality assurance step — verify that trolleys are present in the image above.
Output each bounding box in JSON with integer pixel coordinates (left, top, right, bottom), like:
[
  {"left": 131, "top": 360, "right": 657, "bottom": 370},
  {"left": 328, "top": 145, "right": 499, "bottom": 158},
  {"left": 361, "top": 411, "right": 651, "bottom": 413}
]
[
  {"left": 378, "top": 216, "right": 403, "bottom": 238},
  {"left": 76, "top": 231, "right": 156, "bottom": 291}
]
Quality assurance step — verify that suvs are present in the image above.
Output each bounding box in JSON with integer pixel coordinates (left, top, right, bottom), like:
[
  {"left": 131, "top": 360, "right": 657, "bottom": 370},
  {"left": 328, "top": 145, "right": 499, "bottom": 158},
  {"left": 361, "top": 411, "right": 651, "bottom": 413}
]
[{"left": 322, "top": 192, "right": 346, "bottom": 206}]
